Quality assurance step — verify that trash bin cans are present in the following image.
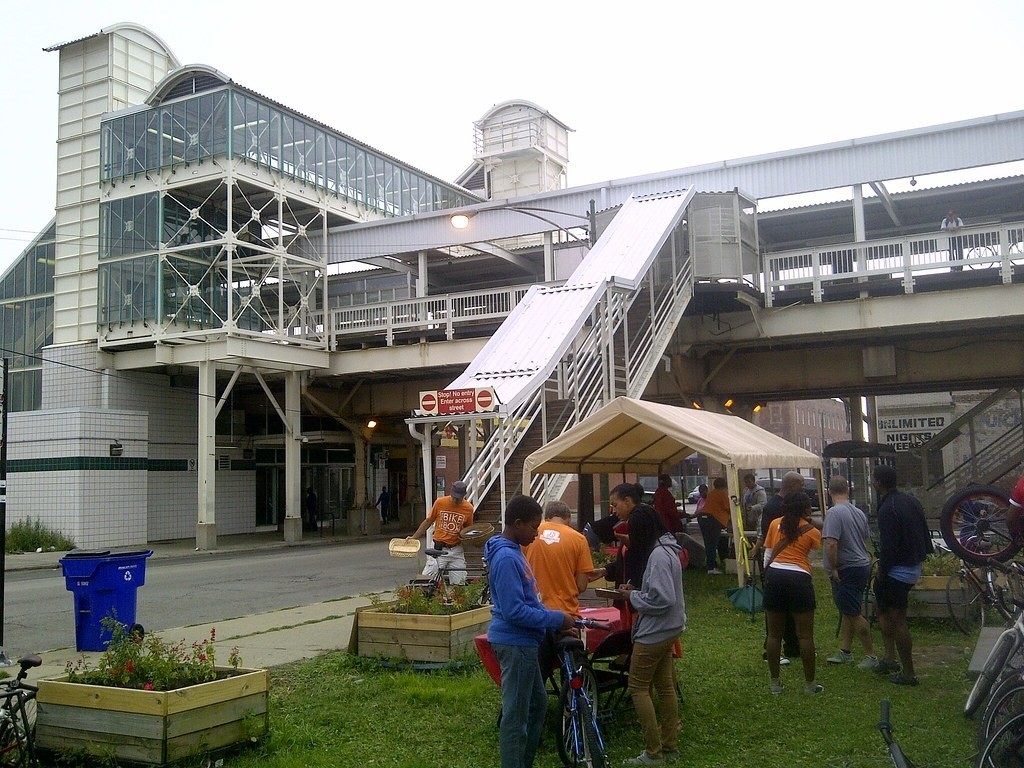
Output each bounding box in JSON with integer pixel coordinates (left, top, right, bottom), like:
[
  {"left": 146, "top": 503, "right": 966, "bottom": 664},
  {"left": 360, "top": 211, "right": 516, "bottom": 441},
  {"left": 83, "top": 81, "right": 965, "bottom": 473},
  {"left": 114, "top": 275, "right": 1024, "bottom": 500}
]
[{"left": 58, "top": 547, "right": 153, "bottom": 653}]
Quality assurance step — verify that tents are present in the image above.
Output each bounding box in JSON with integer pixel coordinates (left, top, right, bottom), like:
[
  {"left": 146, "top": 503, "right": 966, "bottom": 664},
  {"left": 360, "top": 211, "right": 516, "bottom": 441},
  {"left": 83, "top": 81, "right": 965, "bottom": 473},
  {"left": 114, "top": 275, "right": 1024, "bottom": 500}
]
[{"left": 521, "top": 395, "right": 827, "bottom": 587}]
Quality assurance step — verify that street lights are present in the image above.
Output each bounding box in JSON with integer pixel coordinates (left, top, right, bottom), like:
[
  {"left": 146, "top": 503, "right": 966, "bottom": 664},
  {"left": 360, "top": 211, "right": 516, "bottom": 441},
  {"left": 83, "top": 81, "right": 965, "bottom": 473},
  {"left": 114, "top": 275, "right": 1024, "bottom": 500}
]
[{"left": 447, "top": 196, "right": 612, "bottom": 519}]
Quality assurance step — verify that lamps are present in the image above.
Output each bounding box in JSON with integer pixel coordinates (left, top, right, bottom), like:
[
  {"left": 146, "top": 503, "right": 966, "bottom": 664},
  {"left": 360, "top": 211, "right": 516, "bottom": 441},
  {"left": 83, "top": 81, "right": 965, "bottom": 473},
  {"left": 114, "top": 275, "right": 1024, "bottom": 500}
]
[
  {"left": 723, "top": 396, "right": 733, "bottom": 407},
  {"left": 750, "top": 402, "right": 761, "bottom": 412},
  {"left": 110, "top": 443, "right": 123, "bottom": 457},
  {"left": 690, "top": 399, "right": 703, "bottom": 409}
]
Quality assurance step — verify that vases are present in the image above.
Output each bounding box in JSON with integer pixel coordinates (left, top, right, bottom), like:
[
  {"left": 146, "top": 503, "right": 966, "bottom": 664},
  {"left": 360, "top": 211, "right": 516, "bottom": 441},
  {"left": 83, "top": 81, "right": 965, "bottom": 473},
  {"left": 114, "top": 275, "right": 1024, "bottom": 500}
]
[
  {"left": 32, "top": 663, "right": 271, "bottom": 768},
  {"left": 348, "top": 600, "right": 494, "bottom": 664}
]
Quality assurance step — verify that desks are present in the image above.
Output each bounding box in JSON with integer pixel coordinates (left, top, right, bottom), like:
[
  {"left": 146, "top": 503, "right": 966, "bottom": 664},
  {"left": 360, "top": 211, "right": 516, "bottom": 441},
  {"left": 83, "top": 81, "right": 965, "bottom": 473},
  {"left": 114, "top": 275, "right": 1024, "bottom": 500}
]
[{"left": 474, "top": 607, "right": 684, "bottom": 730}]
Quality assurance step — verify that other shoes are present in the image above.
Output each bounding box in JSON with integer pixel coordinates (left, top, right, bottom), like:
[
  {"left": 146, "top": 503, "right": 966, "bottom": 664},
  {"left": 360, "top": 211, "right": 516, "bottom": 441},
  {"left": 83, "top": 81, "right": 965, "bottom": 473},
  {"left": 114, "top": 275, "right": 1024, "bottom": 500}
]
[
  {"left": 827, "top": 649, "right": 854, "bottom": 663},
  {"left": 708, "top": 568, "right": 722, "bottom": 575},
  {"left": 804, "top": 682, "right": 824, "bottom": 695},
  {"left": 875, "top": 660, "right": 901, "bottom": 672},
  {"left": 771, "top": 679, "right": 785, "bottom": 694},
  {"left": 856, "top": 656, "right": 878, "bottom": 668},
  {"left": 628, "top": 752, "right": 663, "bottom": 764},
  {"left": 780, "top": 656, "right": 790, "bottom": 665},
  {"left": 889, "top": 671, "right": 919, "bottom": 685}
]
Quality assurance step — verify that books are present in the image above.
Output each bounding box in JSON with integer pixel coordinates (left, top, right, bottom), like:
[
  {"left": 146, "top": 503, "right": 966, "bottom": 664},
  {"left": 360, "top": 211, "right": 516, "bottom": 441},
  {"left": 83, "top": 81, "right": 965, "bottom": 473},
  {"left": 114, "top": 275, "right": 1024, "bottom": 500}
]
[{"left": 595, "top": 587, "right": 624, "bottom": 601}]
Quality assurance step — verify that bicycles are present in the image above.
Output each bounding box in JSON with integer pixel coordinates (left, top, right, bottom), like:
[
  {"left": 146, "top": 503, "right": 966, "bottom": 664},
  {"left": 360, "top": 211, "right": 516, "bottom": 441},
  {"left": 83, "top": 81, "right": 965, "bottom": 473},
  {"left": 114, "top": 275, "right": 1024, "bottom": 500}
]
[
  {"left": 0, "top": 654, "right": 45, "bottom": 768},
  {"left": 877, "top": 486, "right": 1024, "bottom": 768},
  {"left": 550, "top": 616, "right": 612, "bottom": 768}
]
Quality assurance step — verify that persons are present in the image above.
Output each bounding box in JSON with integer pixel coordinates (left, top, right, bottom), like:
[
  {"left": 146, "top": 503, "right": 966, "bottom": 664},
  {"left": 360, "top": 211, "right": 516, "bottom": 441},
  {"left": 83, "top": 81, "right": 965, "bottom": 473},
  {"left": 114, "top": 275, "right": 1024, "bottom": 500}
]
[
  {"left": 957, "top": 481, "right": 1000, "bottom": 565},
  {"left": 406, "top": 480, "right": 475, "bottom": 586},
  {"left": 301, "top": 486, "right": 318, "bottom": 532},
  {"left": 694, "top": 477, "right": 731, "bottom": 575},
  {"left": 586, "top": 474, "right": 697, "bottom": 764},
  {"left": 1003, "top": 473, "right": 1024, "bottom": 628},
  {"left": 742, "top": 474, "right": 768, "bottom": 530},
  {"left": 941, "top": 210, "right": 964, "bottom": 272},
  {"left": 484, "top": 496, "right": 576, "bottom": 768},
  {"left": 873, "top": 464, "right": 935, "bottom": 686},
  {"left": 519, "top": 500, "right": 595, "bottom": 686},
  {"left": 761, "top": 473, "right": 825, "bottom": 696},
  {"left": 819, "top": 475, "right": 879, "bottom": 669},
  {"left": 374, "top": 486, "right": 389, "bottom": 526}
]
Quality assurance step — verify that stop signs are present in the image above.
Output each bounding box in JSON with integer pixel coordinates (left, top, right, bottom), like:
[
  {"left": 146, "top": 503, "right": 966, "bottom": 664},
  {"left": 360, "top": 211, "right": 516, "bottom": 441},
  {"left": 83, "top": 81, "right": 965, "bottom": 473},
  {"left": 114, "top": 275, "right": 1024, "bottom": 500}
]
[
  {"left": 420, "top": 394, "right": 436, "bottom": 412},
  {"left": 475, "top": 390, "right": 493, "bottom": 408}
]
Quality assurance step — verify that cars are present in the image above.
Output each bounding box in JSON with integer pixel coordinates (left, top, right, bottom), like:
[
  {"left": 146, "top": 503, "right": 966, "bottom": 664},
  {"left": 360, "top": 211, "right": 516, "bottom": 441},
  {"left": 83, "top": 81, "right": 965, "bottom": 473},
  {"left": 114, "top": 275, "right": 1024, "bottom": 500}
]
[
  {"left": 687, "top": 483, "right": 708, "bottom": 504},
  {"left": 751, "top": 475, "right": 856, "bottom": 509}
]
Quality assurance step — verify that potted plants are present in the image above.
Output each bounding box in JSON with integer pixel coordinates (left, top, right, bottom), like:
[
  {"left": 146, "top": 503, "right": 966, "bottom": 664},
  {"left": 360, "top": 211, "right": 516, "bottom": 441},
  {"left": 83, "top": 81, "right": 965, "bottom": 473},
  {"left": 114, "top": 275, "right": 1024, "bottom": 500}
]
[
  {"left": 577, "top": 552, "right": 616, "bottom": 608},
  {"left": 856, "top": 553, "right": 981, "bottom": 620}
]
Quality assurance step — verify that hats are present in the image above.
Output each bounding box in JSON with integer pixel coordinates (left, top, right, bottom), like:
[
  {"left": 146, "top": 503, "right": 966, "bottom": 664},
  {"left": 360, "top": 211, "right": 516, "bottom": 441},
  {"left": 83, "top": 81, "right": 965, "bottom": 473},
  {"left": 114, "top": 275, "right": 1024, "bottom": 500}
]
[{"left": 450, "top": 481, "right": 467, "bottom": 498}]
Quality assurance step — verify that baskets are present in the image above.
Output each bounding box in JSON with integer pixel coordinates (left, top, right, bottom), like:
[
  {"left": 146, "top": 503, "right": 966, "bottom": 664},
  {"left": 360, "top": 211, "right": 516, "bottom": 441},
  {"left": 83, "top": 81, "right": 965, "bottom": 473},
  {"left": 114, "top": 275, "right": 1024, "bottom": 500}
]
[
  {"left": 458, "top": 523, "right": 495, "bottom": 547},
  {"left": 389, "top": 538, "right": 421, "bottom": 558}
]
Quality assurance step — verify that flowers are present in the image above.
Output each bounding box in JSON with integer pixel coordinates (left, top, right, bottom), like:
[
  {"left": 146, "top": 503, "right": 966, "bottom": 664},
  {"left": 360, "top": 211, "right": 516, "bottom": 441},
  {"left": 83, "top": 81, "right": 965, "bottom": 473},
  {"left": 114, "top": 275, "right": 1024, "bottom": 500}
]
[
  {"left": 363, "top": 575, "right": 488, "bottom": 613},
  {"left": 66, "top": 616, "right": 242, "bottom": 692}
]
